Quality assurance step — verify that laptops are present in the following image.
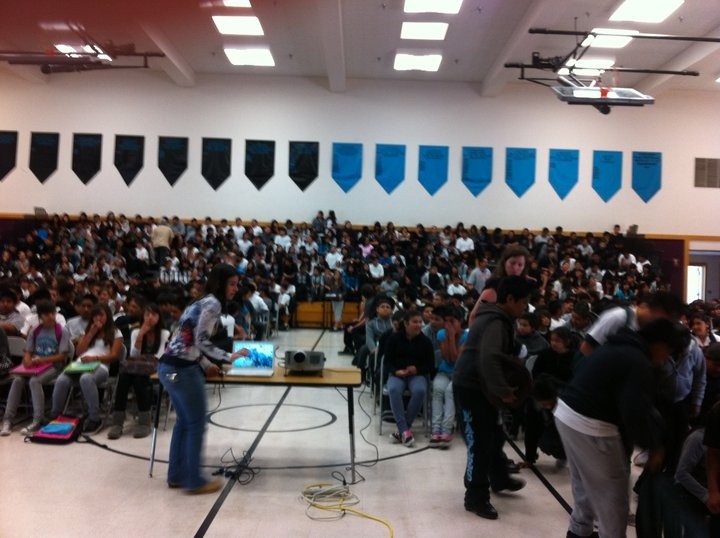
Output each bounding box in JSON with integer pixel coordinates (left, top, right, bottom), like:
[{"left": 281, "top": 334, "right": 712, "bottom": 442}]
[{"left": 226, "top": 341, "right": 275, "bottom": 378}]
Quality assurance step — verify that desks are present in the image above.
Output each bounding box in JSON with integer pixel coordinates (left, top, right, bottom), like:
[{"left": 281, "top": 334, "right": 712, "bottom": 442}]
[{"left": 149, "top": 365, "right": 362, "bottom": 483}]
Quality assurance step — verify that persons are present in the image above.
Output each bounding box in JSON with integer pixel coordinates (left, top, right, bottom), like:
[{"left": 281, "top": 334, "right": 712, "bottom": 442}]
[
  {"left": 675, "top": 401, "right": 720, "bottom": 538},
  {"left": 0, "top": 212, "right": 719, "bottom": 450},
  {"left": 459, "top": 243, "right": 529, "bottom": 473},
  {"left": 629, "top": 322, "right": 692, "bottom": 538},
  {"left": 451, "top": 274, "right": 534, "bottom": 520},
  {"left": 551, "top": 321, "right": 690, "bottom": 538},
  {"left": 158, "top": 263, "right": 253, "bottom": 495}
]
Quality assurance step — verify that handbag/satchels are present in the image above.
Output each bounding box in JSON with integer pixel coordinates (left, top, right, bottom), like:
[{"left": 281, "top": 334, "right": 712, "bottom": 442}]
[
  {"left": 118, "top": 354, "right": 159, "bottom": 375},
  {"left": 24, "top": 412, "right": 82, "bottom": 445}
]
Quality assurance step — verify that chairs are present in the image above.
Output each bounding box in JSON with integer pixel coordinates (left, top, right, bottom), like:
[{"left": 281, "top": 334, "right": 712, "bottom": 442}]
[{"left": 1, "top": 298, "right": 443, "bottom": 438}]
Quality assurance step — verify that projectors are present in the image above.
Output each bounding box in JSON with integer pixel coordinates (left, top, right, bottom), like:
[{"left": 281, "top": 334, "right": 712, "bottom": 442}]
[{"left": 281, "top": 349, "right": 325, "bottom": 376}]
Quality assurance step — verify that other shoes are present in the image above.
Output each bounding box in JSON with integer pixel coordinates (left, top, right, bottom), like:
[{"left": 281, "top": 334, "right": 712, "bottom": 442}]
[
  {"left": 507, "top": 459, "right": 520, "bottom": 473},
  {"left": 492, "top": 476, "right": 526, "bottom": 493},
  {"left": 517, "top": 461, "right": 534, "bottom": 468},
  {"left": 338, "top": 346, "right": 354, "bottom": 355},
  {"left": 464, "top": 501, "right": 498, "bottom": 519},
  {"left": 188, "top": 478, "right": 224, "bottom": 495}
]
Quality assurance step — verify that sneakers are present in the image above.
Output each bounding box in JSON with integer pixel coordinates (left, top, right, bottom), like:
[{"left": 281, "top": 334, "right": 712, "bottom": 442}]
[
  {"left": 428, "top": 434, "right": 454, "bottom": 448},
  {"left": 20, "top": 422, "right": 41, "bottom": 435},
  {"left": 389, "top": 431, "right": 415, "bottom": 447},
  {"left": 0, "top": 420, "right": 13, "bottom": 436},
  {"left": 81, "top": 418, "right": 104, "bottom": 436}
]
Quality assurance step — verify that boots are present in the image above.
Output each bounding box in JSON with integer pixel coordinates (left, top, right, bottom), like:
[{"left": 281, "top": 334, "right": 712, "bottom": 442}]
[
  {"left": 107, "top": 411, "right": 125, "bottom": 439},
  {"left": 133, "top": 411, "right": 151, "bottom": 438}
]
